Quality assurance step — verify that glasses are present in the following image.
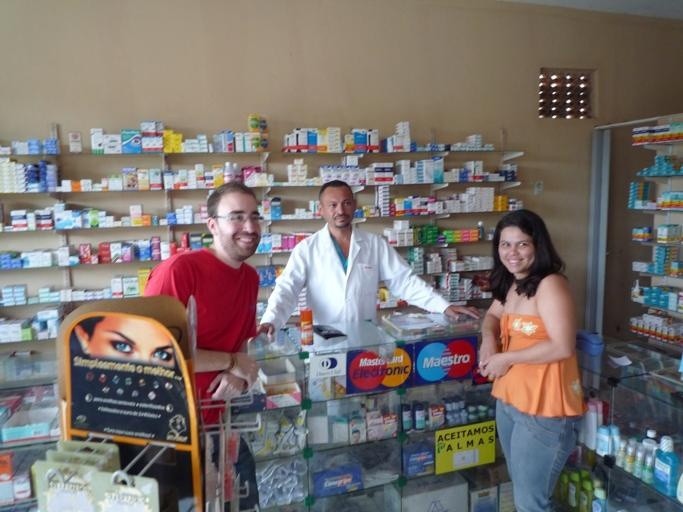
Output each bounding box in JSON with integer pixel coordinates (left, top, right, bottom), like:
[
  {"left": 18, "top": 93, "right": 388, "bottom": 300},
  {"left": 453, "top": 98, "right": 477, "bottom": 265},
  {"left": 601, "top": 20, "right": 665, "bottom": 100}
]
[{"left": 212, "top": 214, "right": 264, "bottom": 225}]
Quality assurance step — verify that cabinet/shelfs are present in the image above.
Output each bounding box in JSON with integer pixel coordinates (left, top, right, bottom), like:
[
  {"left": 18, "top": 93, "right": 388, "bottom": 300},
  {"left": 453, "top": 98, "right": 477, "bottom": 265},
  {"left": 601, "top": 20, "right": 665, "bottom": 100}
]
[
  {"left": 58, "top": 133, "right": 167, "bottom": 312},
  {"left": 620, "top": 113, "right": 682, "bottom": 357},
  {"left": 554, "top": 334, "right": 682, "bottom": 512},
  {"left": 389, "top": 141, "right": 527, "bottom": 308},
  {"left": 270, "top": 133, "right": 390, "bottom": 299},
  {"left": 0, "top": 349, "right": 507, "bottom": 511},
  {"left": 168, "top": 142, "right": 268, "bottom": 294},
  {"left": 0, "top": 129, "right": 65, "bottom": 341}
]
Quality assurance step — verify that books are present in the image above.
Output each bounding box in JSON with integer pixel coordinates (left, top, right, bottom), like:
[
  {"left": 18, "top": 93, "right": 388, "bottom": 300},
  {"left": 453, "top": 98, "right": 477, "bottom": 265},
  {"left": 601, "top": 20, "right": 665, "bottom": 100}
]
[{"left": 382, "top": 313, "right": 436, "bottom": 333}]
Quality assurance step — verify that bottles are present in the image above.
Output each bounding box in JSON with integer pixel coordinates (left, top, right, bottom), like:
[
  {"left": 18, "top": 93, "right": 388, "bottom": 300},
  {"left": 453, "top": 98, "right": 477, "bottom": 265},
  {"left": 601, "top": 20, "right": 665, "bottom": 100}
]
[
  {"left": 223, "top": 160, "right": 241, "bottom": 186},
  {"left": 300, "top": 309, "right": 315, "bottom": 345},
  {"left": 614, "top": 428, "right": 679, "bottom": 498},
  {"left": 477, "top": 221, "right": 484, "bottom": 239},
  {"left": 399, "top": 393, "right": 467, "bottom": 434}
]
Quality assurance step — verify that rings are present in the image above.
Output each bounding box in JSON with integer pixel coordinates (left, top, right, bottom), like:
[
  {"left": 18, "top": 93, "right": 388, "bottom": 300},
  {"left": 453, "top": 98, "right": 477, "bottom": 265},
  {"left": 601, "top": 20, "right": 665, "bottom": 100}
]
[{"left": 478, "top": 361, "right": 481, "bottom": 368}]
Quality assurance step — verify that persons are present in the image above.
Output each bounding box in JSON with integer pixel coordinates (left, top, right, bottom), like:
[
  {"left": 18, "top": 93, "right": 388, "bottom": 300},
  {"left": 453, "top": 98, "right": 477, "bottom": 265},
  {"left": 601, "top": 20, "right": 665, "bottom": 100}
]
[
  {"left": 143, "top": 183, "right": 263, "bottom": 512},
  {"left": 246, "top": 180, "right": 484, "bottom": 468},
  {"left": 478, "top": 210, "right": 589, "bottom": 512},
  {"left": 74, "top": 315, "right": 175, "bottom": 370}
]
